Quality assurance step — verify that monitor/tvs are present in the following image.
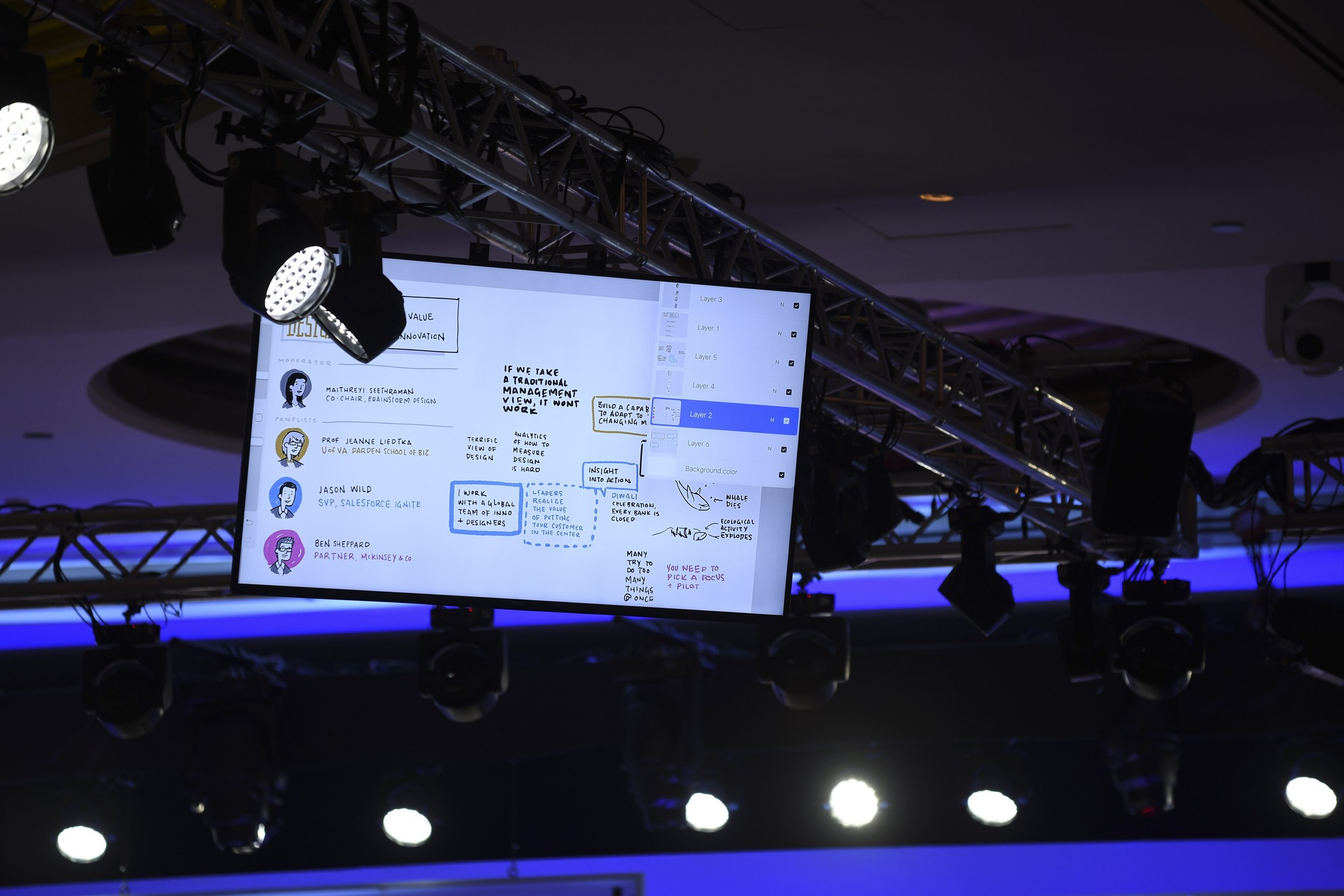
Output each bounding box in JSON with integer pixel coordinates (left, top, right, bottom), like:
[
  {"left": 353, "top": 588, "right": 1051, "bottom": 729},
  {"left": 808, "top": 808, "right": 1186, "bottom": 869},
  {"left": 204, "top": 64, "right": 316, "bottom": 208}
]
[{"left": 230, "top": 250, "right": 815, "bottom": 624}]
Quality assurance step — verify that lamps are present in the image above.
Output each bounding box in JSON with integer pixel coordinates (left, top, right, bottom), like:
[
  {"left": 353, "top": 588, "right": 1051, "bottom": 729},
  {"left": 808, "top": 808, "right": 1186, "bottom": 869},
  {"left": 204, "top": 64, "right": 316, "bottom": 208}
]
[{"left": 0, "top": 69, "right": 1343, "bottom": 863}]
